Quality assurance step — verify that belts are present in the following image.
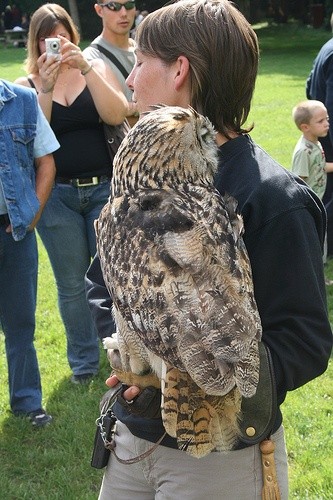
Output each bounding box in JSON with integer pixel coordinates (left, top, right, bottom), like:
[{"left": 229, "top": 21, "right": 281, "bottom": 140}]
[{"left": 56, "top": 173, "right": 112, "bottom": 187}]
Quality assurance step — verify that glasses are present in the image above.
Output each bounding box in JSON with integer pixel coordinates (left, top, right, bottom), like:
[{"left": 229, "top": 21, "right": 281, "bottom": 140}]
[{"left": 100, "top": 0, "right": 136, "bottom": 11}]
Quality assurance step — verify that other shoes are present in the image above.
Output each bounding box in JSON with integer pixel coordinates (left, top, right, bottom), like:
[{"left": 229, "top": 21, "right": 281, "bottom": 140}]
[
  {"left": 71, "top": 374, "right": 94, "bottom": 385},
  {"left": 28, "top": 408, "right": 53, "bottom": 427}
]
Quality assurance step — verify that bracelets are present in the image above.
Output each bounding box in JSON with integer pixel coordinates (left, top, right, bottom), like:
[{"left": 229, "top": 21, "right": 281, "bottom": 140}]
[
  {"left": 41, "top": 87, "right": 55, "bottom": 93},
  {"left": 81, "top": 65, "right": 93, "bottom": 75}
]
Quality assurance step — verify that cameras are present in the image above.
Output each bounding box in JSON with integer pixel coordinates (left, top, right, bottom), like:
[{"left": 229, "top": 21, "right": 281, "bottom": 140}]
[{"left": 45, "top": 38, "right": 63, "bottom": 61}]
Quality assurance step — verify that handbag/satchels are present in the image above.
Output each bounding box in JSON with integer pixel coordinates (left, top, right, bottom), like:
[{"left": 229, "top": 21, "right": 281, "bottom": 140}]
[{"left": 100, "top": 119, "right": 132, "bottom": 165}]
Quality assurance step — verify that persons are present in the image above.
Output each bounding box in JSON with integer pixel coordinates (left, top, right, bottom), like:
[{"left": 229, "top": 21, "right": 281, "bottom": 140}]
[
  {"left": 292, "top": 100, "right": 333, "bottom": 286},
  {"left": 0, "top": 78, "right": 60, "bottom": 427},
  {"left": 91, "top": 0, "right": 333, "bottom": 500},
  {"left": 82, "top": 0, "right": 139, "bottom": 128},
  {"left": 12, "top": 4, "right": 128, "bottom": 386},
  {"left": 306, "top": 14, "right": 333, "bottom": 261}
]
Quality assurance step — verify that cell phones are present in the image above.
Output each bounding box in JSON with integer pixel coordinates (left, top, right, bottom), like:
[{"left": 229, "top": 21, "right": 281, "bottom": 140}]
[{"left": 91, "top": 410, "right": 116, "bottom": 468}]
